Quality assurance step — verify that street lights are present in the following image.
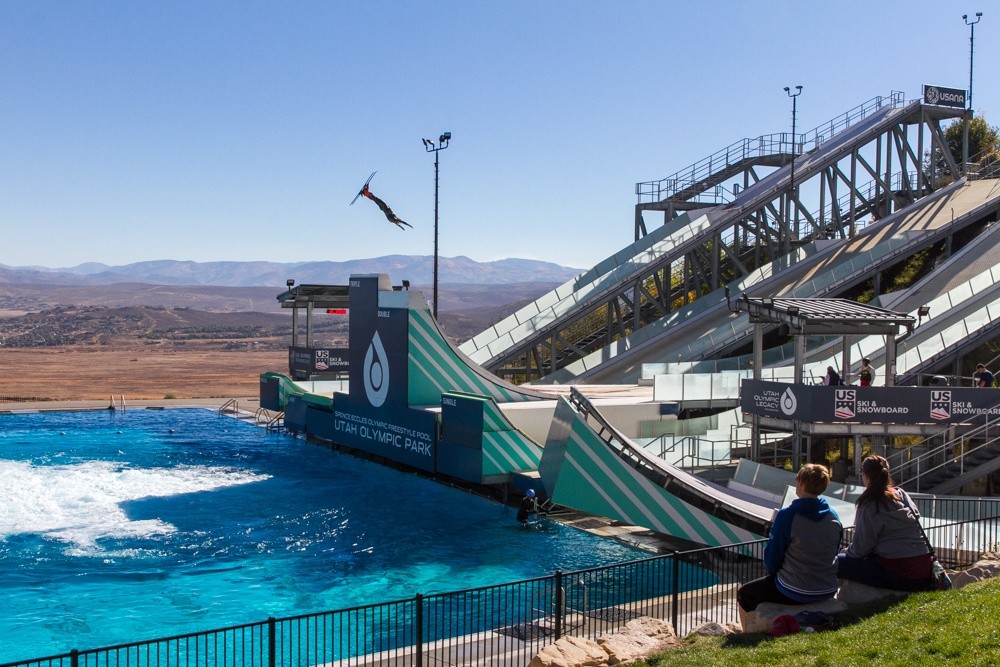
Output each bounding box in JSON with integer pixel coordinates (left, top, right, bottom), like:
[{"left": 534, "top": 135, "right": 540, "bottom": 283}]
[
  {"left": 422, "top": 132, "right": 451, "bottom": 321},
  {"left": 961, "top": 12, "right": 983, "bottom": 111},
  {"left": 784, "top": 85, "right": 803, "bottom": 192}
]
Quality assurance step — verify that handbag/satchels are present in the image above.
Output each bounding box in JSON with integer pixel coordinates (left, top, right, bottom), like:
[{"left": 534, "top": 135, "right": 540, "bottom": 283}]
[{"left": 930, "top": 558, "right": 954, "bottom": 592}]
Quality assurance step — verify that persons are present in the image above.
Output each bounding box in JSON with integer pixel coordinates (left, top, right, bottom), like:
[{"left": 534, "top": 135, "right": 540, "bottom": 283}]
[
  {"left": 515, "top": 489, "right": 550, "bottom": 524},
  {"left": 854, "top": 357, "right": 877, "bottom": 388},
  {"left": 359, "top": 181, "right": 413, "bottom": 230},
  {"left": 831, "top": 455, "right": 926, "bottom": 607},
  {"left": 824, "top": 366, "right": 841, "bottom": 387},
  {"left": 977, "top": 364, "right": 993, "bottom": 387},
  {"left": 734, "top": 464, "right": 846, "bottom": 632}
]
